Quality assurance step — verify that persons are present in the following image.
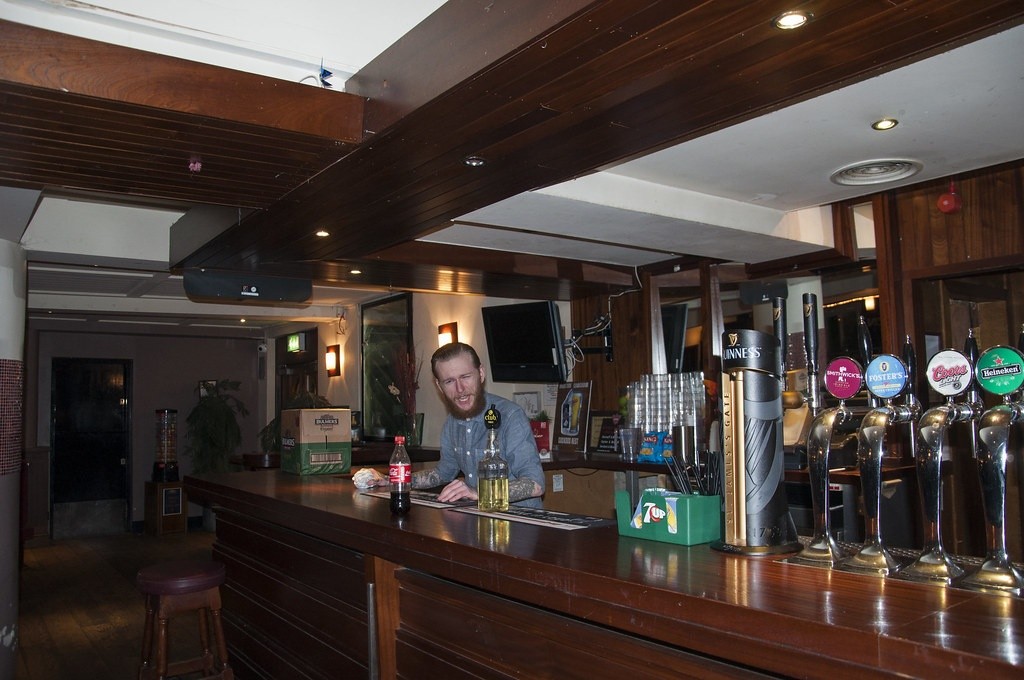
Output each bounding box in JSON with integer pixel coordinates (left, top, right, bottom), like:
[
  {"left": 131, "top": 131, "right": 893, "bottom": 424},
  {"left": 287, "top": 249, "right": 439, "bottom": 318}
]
[{"left": 352, "top": 342, "right": 546, "bottom": 509}]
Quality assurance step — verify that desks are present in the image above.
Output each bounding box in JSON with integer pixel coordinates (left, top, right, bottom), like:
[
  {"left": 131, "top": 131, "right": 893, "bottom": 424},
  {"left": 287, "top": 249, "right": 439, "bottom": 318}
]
[{"left": 144, "top": 479, "right": 187, "bottom": 537}]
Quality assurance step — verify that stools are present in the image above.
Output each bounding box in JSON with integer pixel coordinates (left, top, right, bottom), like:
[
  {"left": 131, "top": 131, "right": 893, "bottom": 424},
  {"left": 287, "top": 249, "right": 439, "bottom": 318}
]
[{"left": 138, "top": 559, "right": 236, "bottom": 680}]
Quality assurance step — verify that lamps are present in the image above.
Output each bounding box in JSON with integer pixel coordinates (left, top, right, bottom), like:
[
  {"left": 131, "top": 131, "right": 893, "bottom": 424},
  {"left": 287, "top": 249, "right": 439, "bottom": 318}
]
[
  {"left": 437, "top": 321, "right": 458, "bottom": 348},
  {"left": 286, "top": 333, "right": 305, "bottom": 352},
  {"left": 326, "top": 345, "right": 341, "bottom": 377}
]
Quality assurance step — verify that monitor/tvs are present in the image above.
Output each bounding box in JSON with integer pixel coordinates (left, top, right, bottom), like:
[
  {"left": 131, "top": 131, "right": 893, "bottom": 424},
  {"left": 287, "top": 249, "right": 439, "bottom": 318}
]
[{"left": 481, "top": 300, "right": 568, "bottom": 384}]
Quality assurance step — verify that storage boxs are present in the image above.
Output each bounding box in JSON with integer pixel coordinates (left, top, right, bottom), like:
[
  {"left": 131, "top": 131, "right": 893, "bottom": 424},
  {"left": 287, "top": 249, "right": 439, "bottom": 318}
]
[{"left": 281, "top": 408, "right": 351, "bottom": 475}]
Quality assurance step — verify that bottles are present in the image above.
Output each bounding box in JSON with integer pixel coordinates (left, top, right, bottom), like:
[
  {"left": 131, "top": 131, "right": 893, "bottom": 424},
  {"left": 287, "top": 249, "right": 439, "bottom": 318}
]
[
  {"left": 478, "top": 429, "right": 509, "bottom": 512},
  {"left": 389, "top": 435, "right": 411, "bottom": 515}
]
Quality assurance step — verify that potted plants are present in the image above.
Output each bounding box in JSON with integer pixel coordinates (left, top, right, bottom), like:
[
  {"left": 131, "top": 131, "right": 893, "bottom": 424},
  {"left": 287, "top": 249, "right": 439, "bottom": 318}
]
[{"left": 181, "top": 392, "right": 250, "bottom": 532}]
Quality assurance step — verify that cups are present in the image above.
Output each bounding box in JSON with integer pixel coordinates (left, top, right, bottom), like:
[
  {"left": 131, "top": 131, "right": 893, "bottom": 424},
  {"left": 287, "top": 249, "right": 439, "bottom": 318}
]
[
  {"left": 403, "top": 413, "right": 424, "bottom": 446},
  {"left": 563, "top": 394, "right": 579, "bottom": 434},
  {"left": 619, "top": 428, "right": 639, "bottom": 460}
]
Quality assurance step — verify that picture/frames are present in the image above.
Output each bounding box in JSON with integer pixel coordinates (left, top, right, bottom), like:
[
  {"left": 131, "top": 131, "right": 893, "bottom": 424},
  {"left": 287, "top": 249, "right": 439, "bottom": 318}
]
[{"left": 587, "top": 411, "right": 624, "bottom": 452}]
